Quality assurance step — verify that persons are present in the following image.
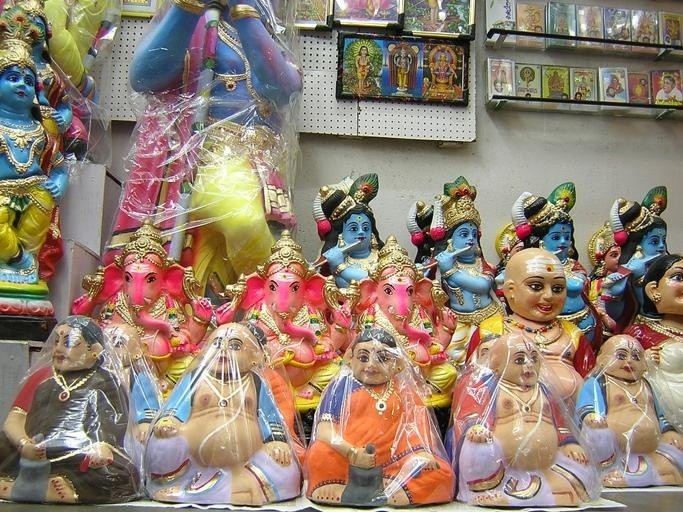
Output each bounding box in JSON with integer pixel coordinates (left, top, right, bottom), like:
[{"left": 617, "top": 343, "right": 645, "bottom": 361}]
[
  {"left": 574, "top": 92, "right": 581, "bottom": 100},
  {"left": 630, "top": 79, "right": 648, "bottom": 102},
  {"left": 21, "top": 1, "right": 74, "bottom": 136},
  {"left": 495, "top": 223, "right": 524, "bottom": 276},
  {"left": 493, "top": 79, "right": 503, "bottom": 92},
  {"left": 608, "top": 76, "right": 623, "bottom": 94},
  {"left": 447, "top": 334, "right": 601, "bottom": 506},
  {"left": 313, "top": 173, "right": 385, "bottom": 288},
  {"left": 143, "top": 323, "right": 303, "bottom": 503},
  {"left": 306, "top": 329, "right": 453, "bottom": 506},
  {"left": 127, "top": 1, "right": 301, "bottom": 303},
  {"left": 624, "top": 254, "right": 682, "bottom": 413},
  {"left": 494, "top": 182, "right": 597, "bottom": 342},
  {"left": 597, "top": 185, "right": 668, "bottom": 343},
  {"left": 654, "top": 76, "right": 682, "bottom": 103},
  {"left": 354, "top": 46, "right": 373, "bottom": 93},
  {"left": 0, "top": 5, "right": 71, "bottom": 285},
  {"left": 2, "top": 315, "right": 143, "bottom": 502},
  {"left": 464, "top": 248, "right": 597, "bottom": 378},
  {"left": 393, "top": 47, "right": 412, "bottom": 89},
  {"left": 604, "top": 88, "right": 625, "bottom": 103},
  {"left": 406, "top": 176, "right": 506, "bottom": 367},
  {"left": 575, "top": 335, "right": 683, "bottom": 487},
  {"left": 583, "top": 222, "right": 622, "bottom": 304},
  {"left": 431, "top": 51, "right": 456, "bottom": 86}
]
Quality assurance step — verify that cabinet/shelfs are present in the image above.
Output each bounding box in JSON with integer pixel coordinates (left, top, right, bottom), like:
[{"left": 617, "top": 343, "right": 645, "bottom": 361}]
[{"left": 0, "top": 162, "right": 124, "bottom": 429}]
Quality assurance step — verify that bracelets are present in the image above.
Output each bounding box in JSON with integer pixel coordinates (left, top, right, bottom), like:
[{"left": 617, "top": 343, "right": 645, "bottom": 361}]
[
  {"left": 591, "top": 414, "right": 597, "bottom": 420},
  {"left": 229, "top": 3, "right": 258, "bottom": 21},
  {"left": 158, "top": 419, "right": 173, "bottom": 425},
  {"left": 17, "top": 439, "right": 28, "bottom": 450},
  {"left": 336, "top": 264, "right": 351, "bottom": 275},
  {"left": 471, "top": 424, "right": 482, "bottom": 430},
  {"left": 348, "top": 447, "right": 358, "bottom": 465},
  {"left": 442, "top": 267, "right": 458, "bottom": 278},
  {"left": 172, "top": 1, "right": 207, "bottom": 16}
]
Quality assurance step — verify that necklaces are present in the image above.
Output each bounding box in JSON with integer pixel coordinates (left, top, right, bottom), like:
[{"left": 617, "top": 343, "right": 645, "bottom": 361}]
[
  {"left": 52, "top": 364, "right": 96, "bottom": 401},
  {"left": 607, "top": 374, "right": 644, "bottom": 404},
  {"left": 506, "top": 317, "right": 563, "bottom": 348},
  {"left": 352, "top": 377, "right": 394, "bottom": 411},
  {"left": 205, "top": 373, "right": 249, "bottom": 407},
  {"left": 0, "top": 120, "right": 46, "bottom": 174},
  {"left": 499, "top": 381, "right": 539, "bottom": 412},
  {"left": 637, "top": 314, "right": 682, "bottom": 341}
]
